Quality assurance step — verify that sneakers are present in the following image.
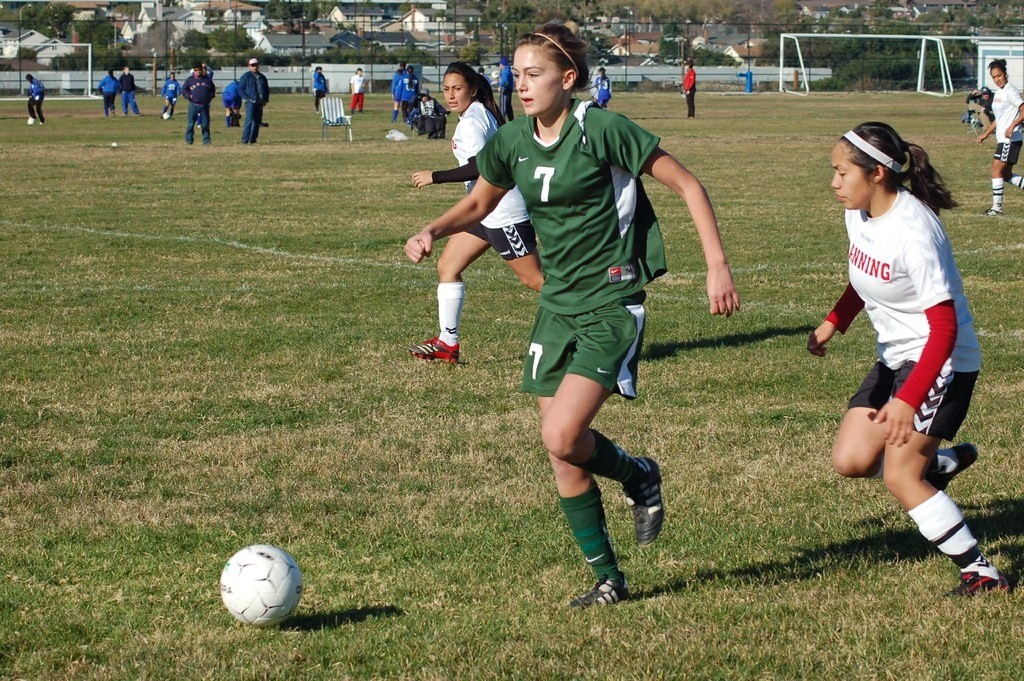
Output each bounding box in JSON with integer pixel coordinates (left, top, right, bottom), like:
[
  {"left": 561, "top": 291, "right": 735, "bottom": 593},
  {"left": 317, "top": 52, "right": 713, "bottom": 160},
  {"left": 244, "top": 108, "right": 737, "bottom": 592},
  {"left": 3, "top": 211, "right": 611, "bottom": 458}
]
[
  {"left": 981, "top": 208, "right": 1003, "bottom": 217},
  {"left": 570, "top": 570, "right": 629, "bottom": 609},
  {"left": 409, "top": 337, "right": 460, "bottom": 366},
  {"left": 942, "top": 568, "right": 1011, "bottom": 598},
  {"left": 926, "top": 442, "right": 978, "bottom": 492},
  {"left": 620, "top": 456, "right": 664, "bottom": 545}
]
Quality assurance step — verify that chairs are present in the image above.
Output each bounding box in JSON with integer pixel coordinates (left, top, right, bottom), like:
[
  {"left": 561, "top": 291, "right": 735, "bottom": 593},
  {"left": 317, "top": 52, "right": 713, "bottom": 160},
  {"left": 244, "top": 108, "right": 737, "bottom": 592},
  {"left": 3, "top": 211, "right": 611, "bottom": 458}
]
[{"left": 318, "top": 97, "right": 353, "bottom": 142}]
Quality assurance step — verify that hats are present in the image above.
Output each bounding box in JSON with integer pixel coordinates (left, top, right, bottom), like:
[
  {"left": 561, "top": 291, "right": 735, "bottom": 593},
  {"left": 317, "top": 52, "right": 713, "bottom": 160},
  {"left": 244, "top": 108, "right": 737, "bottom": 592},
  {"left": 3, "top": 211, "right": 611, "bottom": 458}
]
[
  {"left": 249, "top": 58, "right": 258, "bottom": 65},
  {"left": 981, "top": 86, "right": 991, "bottom": 93}
]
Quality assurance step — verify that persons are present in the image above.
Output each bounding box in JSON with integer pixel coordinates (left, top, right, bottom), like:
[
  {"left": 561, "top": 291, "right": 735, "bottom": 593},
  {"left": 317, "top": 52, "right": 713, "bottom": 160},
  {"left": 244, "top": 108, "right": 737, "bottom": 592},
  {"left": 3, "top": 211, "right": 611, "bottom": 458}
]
[
  {"left": 119, "top": 66, "right": 141, "bottom": 116},
  {"left": 403, "top": 21, "right": 740, "bottom": 611},
  {"left": 160, "top": 58, "right": 270, "bottom": 145},
  {"left": 98, "top": 67, "right": 119, "bottom": 119},
  {"left": 349, "top": 68, "right": 366, "bottom": 114},
  {"left": 313, "top": 66, "right": 326, "bottom": 113},
  {"left": 683, "top": 61, "right": 696, "bottom": 118},
  {"left": 590, "top": 67, "right": 612, "bottom": 111},
  {"left": 806, "top": 122, "right": 1009, "bottom": 601},
  {"left": 25, "top": 74, "right": 45, "bottom": 126},
  {"left": 391, "top": 57, "right": 515, "bottom": 140},
  {"left": 408, "top": 60, "right": 546, "bottom": 362},
  {"left": 966, "top": 58, "right": 1024, "bottom": 216}
]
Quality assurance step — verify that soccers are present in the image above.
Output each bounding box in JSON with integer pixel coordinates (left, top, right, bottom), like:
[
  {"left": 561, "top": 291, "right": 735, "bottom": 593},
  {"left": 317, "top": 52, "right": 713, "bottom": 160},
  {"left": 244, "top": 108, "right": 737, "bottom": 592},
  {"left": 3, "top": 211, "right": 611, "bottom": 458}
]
[
  {"left": 163, "top": 113, "right": 171, "bottom": 119},
  {"left": 220, "top": 545, "right": 304, "bottom": 625},
  {"left": 27, "top": 118, "right": 35, "bottom": 125}
]
